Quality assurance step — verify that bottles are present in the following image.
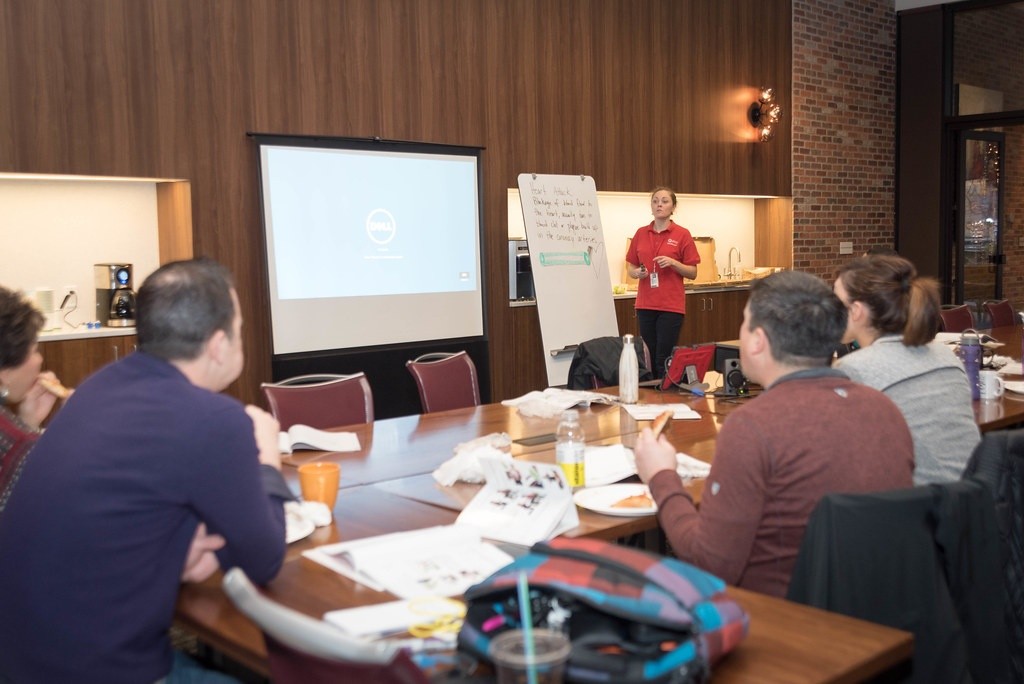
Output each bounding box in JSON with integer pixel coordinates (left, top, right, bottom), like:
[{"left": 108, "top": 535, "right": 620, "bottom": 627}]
[
  {"left": 618, "top": 334, "right": 640, "bottom": 404},
  {"left": 556, "top": 409, "right": 586, "bottom": 487},
  {"left": 959, "top": 329, "right": 981, "bottom": 400}
]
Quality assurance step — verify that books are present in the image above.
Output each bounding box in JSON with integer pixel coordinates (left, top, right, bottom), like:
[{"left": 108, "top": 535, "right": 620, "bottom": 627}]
[
  {"left": 279, "top": 425, "right": 362, "bottom": 454},
  {"left": 456, "top": 460, "right": 580, "bottom": 546},
  {"left": 933, "top": 333, "right": 998, "bottom": 345},
  {"left": 501, "top": 388, "right": 620, "bottom": 410}
]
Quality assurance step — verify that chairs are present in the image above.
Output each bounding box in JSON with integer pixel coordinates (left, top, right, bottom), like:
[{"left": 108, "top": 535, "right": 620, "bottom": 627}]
[
  {"left": 260, "top": 371, "right": 374, "bottom": 431},
  {"left": 938, "top": 304, "right": 976, "bottom": 332},
  {"left": 405, "top": 350, "right": 480, "bottom": 414},
  {"left": 982, "top": 298, "right": 1016, "bottom": 328}
]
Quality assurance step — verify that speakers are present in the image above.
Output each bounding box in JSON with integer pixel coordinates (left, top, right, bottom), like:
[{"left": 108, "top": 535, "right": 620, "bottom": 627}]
[{"left": 723, "top": 358, "right": 748, "bottom": 397}]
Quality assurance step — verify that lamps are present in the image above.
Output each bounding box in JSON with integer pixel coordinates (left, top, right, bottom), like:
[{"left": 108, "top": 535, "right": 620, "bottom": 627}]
[{"left": 748, "top": 85, "right": 783, "bottom": 143}]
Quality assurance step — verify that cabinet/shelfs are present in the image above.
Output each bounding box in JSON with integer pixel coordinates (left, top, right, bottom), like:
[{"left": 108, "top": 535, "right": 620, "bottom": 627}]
[
  {"left": 6, "top": 335, "right": 141, "bottom": 430},
  {"left": 614, "top": 290, "right": 750, "bottom": 347}
]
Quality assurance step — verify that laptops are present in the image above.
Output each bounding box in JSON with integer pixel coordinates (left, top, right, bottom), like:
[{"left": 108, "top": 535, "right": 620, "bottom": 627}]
[{"left": 638, "top": 342, "right": 715, "bottom": 390}]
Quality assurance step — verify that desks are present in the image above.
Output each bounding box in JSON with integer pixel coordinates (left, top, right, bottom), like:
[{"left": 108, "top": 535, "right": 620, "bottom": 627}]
[{"left": 171, "top": 325, "right": 1024, "bottom": 684}]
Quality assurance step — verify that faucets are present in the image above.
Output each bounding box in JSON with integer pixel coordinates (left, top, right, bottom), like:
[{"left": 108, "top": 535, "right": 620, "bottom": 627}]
[{"left": 723, "top": 246, "right": 742, "bottom": 280}]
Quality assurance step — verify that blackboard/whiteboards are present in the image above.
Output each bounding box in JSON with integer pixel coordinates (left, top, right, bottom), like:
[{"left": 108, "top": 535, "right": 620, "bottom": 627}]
[{"left": 517, "top": 173, "right": 619, "bottom": 387}]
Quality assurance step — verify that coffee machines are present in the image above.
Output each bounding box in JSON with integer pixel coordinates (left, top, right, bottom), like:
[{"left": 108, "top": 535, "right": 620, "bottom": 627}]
[{"left": 93, "top": 263, "right": 137, "bottom": 326}]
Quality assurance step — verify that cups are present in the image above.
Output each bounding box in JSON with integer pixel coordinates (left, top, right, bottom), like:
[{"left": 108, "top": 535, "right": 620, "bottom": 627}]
[
  {"left": 415, "top": 653, "right": 478, "bottom": 684},
  {"left": 488, "top": 630, "right": 569, "bottom": 683},
  {"left": 297, "top": 462, "right": 339, "bottom": 511},
  {"left": 978, "top": 370, "right": 1005, "bottom": 399}
]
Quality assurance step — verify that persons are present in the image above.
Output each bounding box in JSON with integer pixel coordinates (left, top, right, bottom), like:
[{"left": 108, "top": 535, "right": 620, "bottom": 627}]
[
  {"left": 830, "top": 255, "right": 981, "bottom": 486},
  {"left": 491, "top": 464, "right": 563, "bottom": 509},
  {"left": 625, "top": 187, "right": 701, "bottom": 380},
  {"left": 0, "top": 288, "right": 74, "bottom": 505},
  {"left": 634, "top": 268, "right": 915, "bottom": 598},
  {"left": 0, "top": 258, "right": 303, "bottom": 683}
]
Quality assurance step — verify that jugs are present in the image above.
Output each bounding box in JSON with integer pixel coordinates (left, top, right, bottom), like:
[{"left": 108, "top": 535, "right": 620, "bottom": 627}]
[{"left": 110, "top": 288, "right": 137, "bottom": 319}]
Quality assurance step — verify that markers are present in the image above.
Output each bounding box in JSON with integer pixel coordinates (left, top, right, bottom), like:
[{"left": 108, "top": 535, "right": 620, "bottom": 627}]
[{"left": 641, "top": 264, "right": 645, "bottom": 272}]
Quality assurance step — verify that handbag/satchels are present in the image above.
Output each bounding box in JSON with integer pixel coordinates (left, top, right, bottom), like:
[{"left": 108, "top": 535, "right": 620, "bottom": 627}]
[{"left": 454, "top": 539, "right": 749, "bottom": 684}]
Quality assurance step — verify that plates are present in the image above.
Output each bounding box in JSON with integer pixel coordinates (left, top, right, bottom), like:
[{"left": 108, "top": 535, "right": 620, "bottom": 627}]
[{"left": 574, "top": 482, "right": 658, "bottom": 517}]
